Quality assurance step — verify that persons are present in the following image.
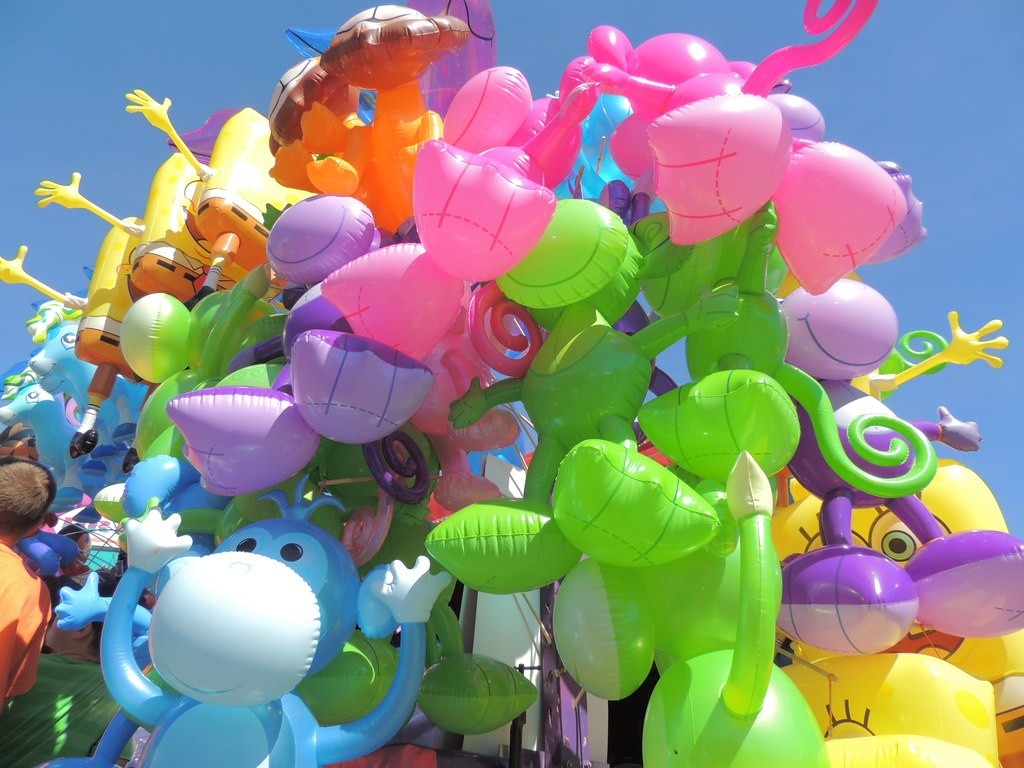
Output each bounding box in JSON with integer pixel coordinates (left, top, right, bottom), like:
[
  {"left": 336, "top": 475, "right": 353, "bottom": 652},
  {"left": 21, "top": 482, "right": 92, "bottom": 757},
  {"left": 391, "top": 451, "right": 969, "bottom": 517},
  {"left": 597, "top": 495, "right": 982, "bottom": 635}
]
[
  {"left": 0, "top": 458, "right": 54, "bottom": 708},
  {"left": 0, "top": 568, "right": 137, "bottom": 768}
]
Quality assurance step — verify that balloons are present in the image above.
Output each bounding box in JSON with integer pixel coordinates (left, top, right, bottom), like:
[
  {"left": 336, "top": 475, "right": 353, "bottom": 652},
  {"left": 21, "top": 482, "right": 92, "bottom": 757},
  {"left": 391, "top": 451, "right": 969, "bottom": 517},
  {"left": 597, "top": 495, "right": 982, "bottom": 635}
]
[{"left": 2, "top": 0, "right": 1024, "bottom": 768}]
